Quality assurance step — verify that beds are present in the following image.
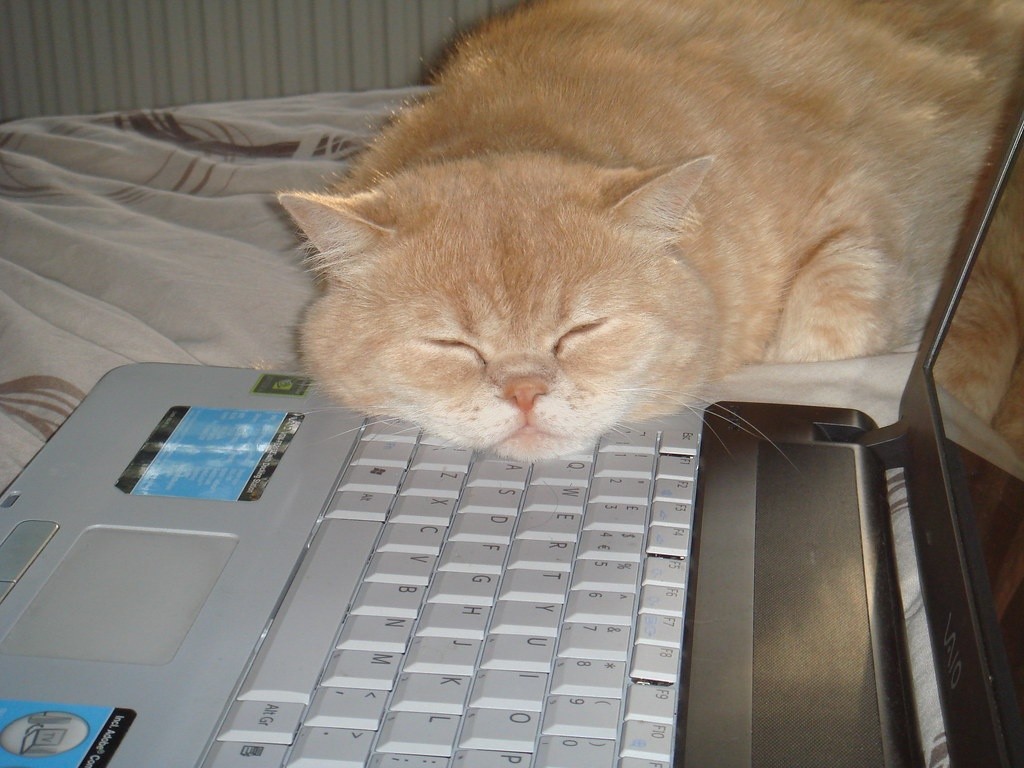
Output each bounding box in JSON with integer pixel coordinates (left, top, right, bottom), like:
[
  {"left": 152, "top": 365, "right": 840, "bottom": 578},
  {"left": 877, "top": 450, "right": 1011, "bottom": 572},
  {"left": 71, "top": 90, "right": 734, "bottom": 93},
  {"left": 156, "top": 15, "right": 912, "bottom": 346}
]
[{"left": 0, "top": 84, "right": 922, "bottom": 481}]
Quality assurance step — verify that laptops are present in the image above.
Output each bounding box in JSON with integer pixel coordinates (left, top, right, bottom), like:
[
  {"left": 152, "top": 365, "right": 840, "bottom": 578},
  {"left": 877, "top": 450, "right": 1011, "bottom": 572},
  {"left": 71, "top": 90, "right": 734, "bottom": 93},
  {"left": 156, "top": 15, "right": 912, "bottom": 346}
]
[{"left": 0, "top": 69, "right": 1024, "bottom": 768}]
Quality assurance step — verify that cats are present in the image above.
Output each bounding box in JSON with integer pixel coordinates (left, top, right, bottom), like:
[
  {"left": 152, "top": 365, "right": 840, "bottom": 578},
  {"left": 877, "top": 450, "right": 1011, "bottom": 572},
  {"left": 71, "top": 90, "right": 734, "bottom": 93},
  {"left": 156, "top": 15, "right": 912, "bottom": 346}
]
[
  {"left": 934, "top": 142, "right": 1024, "bottom": 458},
  {"left": 274, "top": 1, "right": 1024, "bottom": 462}
]
